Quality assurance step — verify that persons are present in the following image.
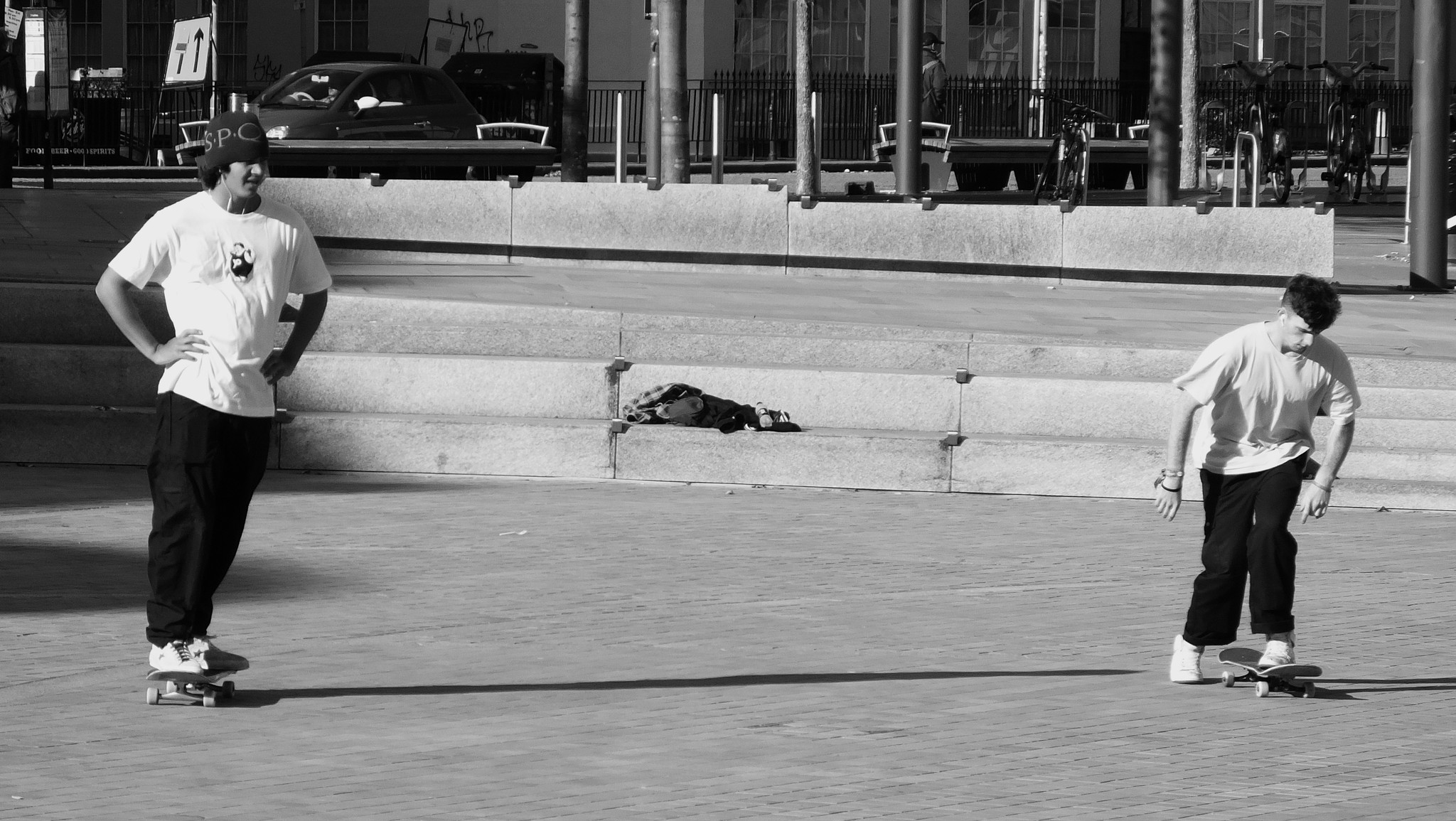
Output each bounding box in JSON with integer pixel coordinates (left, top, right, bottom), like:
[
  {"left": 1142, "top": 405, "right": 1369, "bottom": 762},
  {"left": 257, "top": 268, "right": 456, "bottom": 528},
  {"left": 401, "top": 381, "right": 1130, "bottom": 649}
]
[
  {"left": 93, "top": 109, "right": 334, "bottom": 679},
  {"left": 1152, "top": 270, "right": 1361, "bottom": 686}
]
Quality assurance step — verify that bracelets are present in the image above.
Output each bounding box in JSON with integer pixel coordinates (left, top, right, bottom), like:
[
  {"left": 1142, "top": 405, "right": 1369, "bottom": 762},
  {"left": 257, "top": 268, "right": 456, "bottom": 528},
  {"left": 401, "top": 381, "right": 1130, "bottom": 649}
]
[
  {"left": 1153, "top": 468, "right": 1185, "bottom": 488},
  {"left": 1313, "top": 480, "right": 1333, "bottom": 493},
  {"left": 1161, "top": 480, "right": 1182, "bottom": 492}
]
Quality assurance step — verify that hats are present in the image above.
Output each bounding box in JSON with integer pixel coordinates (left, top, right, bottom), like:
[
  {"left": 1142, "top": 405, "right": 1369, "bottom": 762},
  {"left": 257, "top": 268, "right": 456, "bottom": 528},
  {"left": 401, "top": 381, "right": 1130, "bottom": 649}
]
[{"left": 204, "top": 109, "right": 270, "bottom": 166}]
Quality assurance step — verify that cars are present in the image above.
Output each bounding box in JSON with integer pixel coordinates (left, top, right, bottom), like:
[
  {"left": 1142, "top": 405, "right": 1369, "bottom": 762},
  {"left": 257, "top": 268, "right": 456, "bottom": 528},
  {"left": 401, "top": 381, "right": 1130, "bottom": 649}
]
[{"left": 226, "top": 60, "right": 498, "bottom": 182}]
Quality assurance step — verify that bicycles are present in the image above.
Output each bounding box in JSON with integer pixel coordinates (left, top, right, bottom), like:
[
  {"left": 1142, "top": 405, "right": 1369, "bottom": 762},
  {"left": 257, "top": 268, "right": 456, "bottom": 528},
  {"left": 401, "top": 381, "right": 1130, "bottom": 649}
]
[
  {"left": 1221, "top": 58, "right": 1302, "bottom": 206},
  {"left": 1306, "top": 58, "right": 1391, "bottom": 203},
  {"left": 1026, "top": 88, "right": 1115, "bottom": 208}
]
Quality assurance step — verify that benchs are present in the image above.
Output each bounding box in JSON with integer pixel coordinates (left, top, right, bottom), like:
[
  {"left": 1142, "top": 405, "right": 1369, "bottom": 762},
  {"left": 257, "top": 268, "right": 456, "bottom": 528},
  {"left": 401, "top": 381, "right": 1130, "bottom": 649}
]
[
  {"left": 172, "top": 111, "right": 559, "bottom": 180},
  {"left": 864, "top": 118, "right": 1199, "bottom": 199}
]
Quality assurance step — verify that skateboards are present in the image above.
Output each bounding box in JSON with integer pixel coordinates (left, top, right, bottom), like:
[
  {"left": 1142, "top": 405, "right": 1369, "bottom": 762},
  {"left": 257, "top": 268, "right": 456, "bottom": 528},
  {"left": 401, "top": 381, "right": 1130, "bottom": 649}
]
[
  {"left": 1216, "top": 647, "right": 1324, "bottom": 699},
  {"left": 142, "top": 658, "right": 239, "bottom": 708}
]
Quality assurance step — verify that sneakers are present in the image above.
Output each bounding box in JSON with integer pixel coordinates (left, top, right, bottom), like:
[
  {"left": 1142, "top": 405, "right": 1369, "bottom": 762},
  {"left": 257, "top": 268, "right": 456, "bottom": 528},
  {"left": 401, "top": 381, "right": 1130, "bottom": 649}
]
[
  {"left": 1170, "top": 633, "right": 1206, "bottom": 683},
  {"left": 1257, "top": 631, "right": 1300, "bottom": 669},
  {"left": 148, "top": 639, "right": 203, "bottom": 675},
  {"left": 189, "top": 634, "right": 250, "bottom": 674}
]
